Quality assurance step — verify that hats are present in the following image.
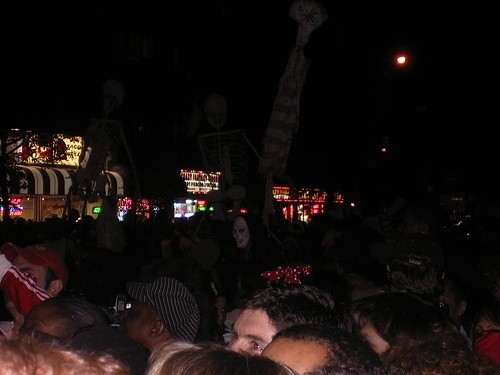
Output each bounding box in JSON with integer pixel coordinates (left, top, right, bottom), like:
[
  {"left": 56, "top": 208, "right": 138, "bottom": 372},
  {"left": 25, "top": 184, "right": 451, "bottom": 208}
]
[
  {"left": 0, "top": 242, "right": 71, "bottom": 296},
  {"left": 125, "top": 277, "right": 201, "bottom": 345}
]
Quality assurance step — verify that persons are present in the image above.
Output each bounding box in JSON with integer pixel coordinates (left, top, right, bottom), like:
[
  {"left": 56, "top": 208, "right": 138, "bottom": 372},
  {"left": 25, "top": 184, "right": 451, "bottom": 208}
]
[{"left": 1, "top": 200, "right": 500, "bottom": 375}]
